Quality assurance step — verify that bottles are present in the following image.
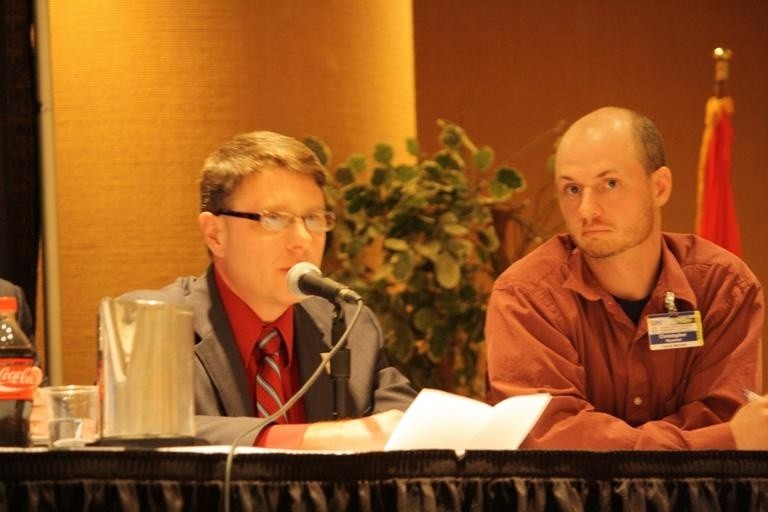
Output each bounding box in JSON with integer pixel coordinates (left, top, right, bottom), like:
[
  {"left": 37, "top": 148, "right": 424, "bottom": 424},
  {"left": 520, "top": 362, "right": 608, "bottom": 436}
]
[{"left": 0, "top": 296, "right": 35, "bottom": 448}]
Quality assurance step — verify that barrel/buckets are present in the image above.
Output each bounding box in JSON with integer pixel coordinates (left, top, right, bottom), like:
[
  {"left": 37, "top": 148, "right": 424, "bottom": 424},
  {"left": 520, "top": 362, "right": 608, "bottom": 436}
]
[{"left": 92, "top": 294, "right": 201, "bottom": 444}]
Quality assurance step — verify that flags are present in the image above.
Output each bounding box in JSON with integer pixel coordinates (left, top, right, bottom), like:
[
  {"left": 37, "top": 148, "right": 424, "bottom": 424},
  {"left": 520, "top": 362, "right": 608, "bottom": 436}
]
[{"left": 693, "top": 96, "right": 741, "bottom": 257}]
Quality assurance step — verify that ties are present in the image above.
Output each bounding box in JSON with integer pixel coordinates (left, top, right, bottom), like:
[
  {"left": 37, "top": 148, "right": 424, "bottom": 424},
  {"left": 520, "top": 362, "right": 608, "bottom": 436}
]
[{"left": 254, "top": 325, "right": 289, "bottom": 425}]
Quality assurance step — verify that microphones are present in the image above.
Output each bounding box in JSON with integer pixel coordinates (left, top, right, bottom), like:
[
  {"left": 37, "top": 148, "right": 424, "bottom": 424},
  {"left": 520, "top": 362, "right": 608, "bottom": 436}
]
[{"left": 285, "top": 261, "right": 364, "bottom": 305}]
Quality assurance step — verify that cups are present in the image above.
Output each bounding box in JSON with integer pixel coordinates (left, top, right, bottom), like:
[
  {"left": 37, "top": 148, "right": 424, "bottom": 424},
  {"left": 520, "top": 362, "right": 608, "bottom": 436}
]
[{"left": 37, "top": 384, "right": 100, "bottom": 447}]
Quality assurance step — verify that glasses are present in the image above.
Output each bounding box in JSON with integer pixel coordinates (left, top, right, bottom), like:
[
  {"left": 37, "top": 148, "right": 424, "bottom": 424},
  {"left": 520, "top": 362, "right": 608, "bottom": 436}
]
[{"left": 218, "top": 208, "right": 335, "bottom": 232}]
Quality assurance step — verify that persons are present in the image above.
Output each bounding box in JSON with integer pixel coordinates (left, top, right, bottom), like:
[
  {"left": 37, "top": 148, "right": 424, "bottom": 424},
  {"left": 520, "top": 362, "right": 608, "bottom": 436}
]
[
  {"left": 480, "top": 103, "right": 767, "bottom": 457},
  {"left": 113, "top": 126, "right": 422, "bottom": 454}
]
